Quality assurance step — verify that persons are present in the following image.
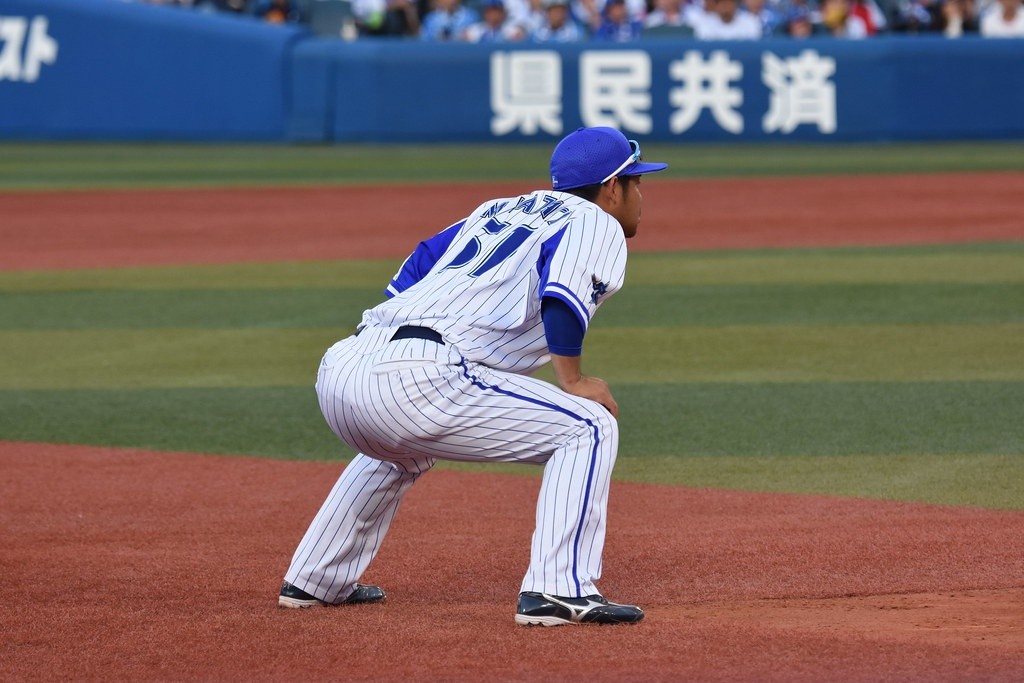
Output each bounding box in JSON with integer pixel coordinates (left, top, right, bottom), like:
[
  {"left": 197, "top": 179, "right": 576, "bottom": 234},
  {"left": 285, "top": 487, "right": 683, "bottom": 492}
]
[
  {"left": 277, "top": 127, "right": 669, "bottom": 630},
  {"left": 196, "top": 0, "right": 1022, "bottom": 47}
]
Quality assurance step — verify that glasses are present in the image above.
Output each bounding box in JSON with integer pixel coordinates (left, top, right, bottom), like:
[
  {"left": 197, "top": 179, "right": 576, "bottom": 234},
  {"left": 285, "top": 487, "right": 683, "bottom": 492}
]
[{"left": 600, "top": 140, "right": 642, "bottom": 185}]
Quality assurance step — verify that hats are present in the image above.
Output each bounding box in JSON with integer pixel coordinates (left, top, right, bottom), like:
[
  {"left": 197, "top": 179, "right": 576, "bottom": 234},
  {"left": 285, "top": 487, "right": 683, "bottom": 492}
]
[{"left": 550, "top": 127, "right": 668, "bottom": 191}]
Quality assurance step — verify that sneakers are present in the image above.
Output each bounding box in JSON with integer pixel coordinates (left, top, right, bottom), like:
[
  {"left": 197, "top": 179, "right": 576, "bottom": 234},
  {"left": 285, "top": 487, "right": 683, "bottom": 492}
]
[
  {"left": 515, "top": 591, "right": 644, "bottom": 625},
  {"left": 278, "top": 580, "right": 386, "bottom": 609}
]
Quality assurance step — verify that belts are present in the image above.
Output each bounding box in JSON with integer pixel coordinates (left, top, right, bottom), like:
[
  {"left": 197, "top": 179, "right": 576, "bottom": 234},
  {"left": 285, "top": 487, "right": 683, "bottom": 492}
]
[{"left": 354, "top": 325, "right": 445, "bottom": 345}]
